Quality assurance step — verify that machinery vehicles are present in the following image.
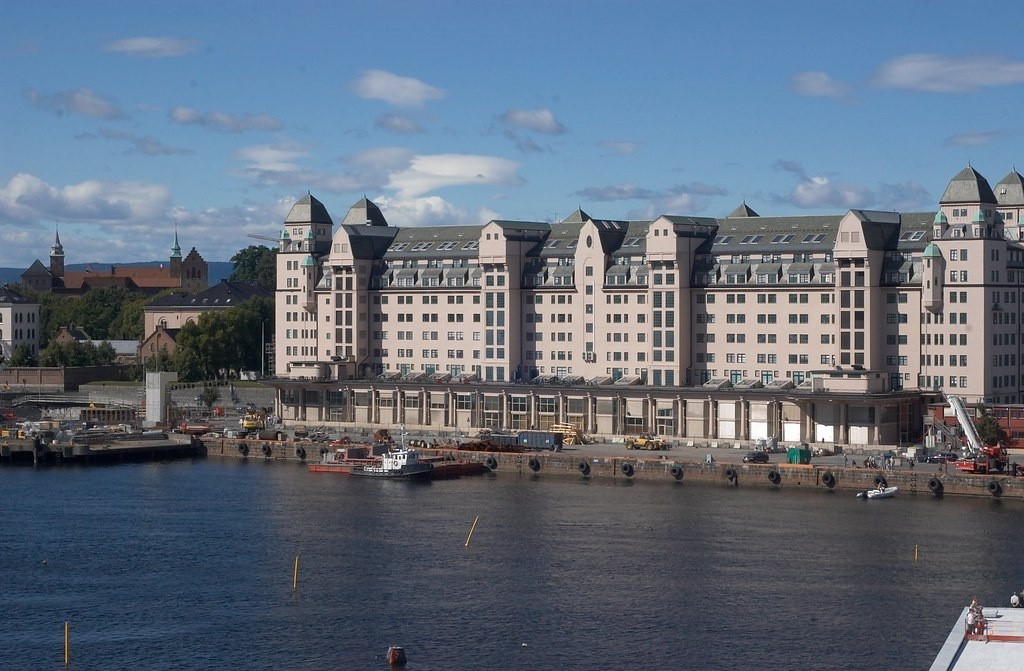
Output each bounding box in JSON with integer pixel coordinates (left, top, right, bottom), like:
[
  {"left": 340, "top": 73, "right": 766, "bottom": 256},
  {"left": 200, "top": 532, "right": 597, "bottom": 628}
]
[{"left": 947, "top": 395, "right": 1010, "bottom": 472}]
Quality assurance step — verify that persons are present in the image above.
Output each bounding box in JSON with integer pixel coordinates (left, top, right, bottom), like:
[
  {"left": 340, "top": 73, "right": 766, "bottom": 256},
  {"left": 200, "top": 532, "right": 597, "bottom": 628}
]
[
  {"left": 167, "top": 400, "right": 391, "bottom": 444},
  {"left": 843, "top": 440, "right": 985, "bottom": 472},
  {"left": 876, "top": 482, "right": 885, "bottom": 494}
]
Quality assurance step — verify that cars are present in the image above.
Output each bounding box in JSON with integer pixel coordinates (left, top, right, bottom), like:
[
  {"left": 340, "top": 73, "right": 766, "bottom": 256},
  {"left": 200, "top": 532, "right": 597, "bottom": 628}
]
[
  {"left": 916, "top": 448, "right": 958, "bottom": 464},
  {"left": 741, "top": 451, "right": 769, "bottom": 463}
]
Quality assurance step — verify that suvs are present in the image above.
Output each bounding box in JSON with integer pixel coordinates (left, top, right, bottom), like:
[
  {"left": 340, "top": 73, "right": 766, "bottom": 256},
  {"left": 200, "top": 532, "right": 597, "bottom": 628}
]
[{"left": 625, "top": 430, "right": 667, "bottom": 451}]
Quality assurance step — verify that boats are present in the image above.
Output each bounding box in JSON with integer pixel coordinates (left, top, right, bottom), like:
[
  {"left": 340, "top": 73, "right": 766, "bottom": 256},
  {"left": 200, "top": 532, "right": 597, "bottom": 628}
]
[
  {"left": 306, "top": 422, "right": 485, "bottom": 477},
  {"left": 857, "top": 485, "right": 899, "bottom": 501}
]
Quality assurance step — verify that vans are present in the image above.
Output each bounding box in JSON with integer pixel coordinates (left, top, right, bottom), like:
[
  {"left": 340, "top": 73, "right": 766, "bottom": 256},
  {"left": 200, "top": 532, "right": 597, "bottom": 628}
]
[{"left": 242, "top": 415, "right": 258, "bottom": 428}]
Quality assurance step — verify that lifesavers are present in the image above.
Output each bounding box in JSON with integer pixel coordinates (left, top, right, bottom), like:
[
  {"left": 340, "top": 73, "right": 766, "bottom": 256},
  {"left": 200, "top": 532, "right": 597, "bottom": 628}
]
[
  {"left": 528, "top": 459, "right": 537, "bottom": 469},
  {"left": 486, "top": 457, "right": 496, "bottom": 467},
  {"left": 622, "top": 464, "right": 631, "bottom": 475},
  {"left": 670, "top": 467, "right": 681, "bottom": 477},
  {"left": 822, "top": 473, "right": 833, "bottom": 485},
  {"left": 768, "top": 470, "right": 778, "bottom": 482},
  {"left": 577, "top": 462, "right": 588, "bottom": 473},
  {"left": 928, "top": 478, "right": 940, "bottom": 491},
  {"left": 988, "top": 482, "right": 999, "bottom": 493},
  {"left": 725, "top": 469, "right": 734, "bottom": 479},
  {"left": 873, "top": 476, "right": 884, "bottom": 488}
]
